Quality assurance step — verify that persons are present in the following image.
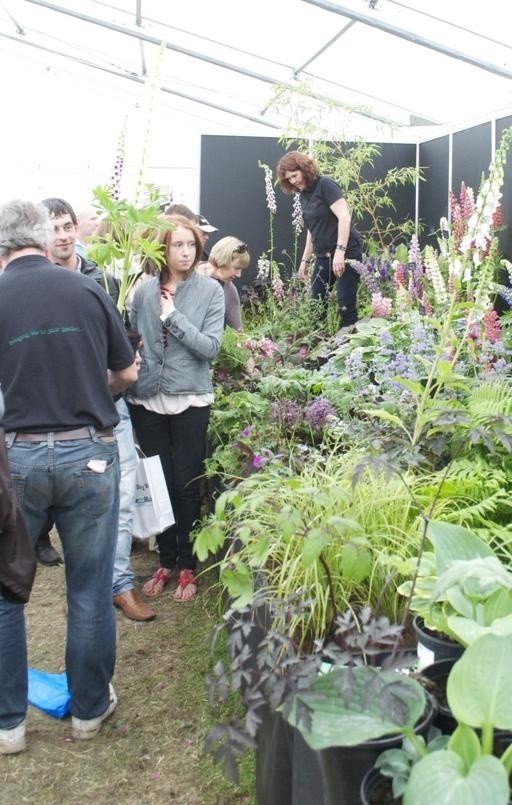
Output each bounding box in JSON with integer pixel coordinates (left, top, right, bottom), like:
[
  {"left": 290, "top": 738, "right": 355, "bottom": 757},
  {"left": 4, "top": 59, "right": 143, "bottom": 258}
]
[
  {"left": 0, "top": 199, "right": 137, "bottom": 754},
  {"left": 0, "top": 199, "right": 251, "bottom": 617},
  {"left": 278, "top": 151, "right": 362, "bottom": 329}
]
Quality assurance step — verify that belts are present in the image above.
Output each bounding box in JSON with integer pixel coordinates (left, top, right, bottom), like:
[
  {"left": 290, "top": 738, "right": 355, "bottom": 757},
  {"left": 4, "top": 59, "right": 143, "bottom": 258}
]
[{"left": 5, "top": 424, "right": 114, "bottom": 444}]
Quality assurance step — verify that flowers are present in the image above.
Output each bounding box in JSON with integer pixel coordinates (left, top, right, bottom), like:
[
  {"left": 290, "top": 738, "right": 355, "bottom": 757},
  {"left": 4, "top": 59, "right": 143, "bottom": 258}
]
[{"left": 174, "top": 69, "right": 511, "bottom": 804}]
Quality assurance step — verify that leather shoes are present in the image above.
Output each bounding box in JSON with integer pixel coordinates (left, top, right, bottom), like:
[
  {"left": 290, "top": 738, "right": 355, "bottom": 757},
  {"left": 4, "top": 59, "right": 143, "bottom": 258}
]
[
  {"left": 110, "top": 585, "right": 159, "bottom": 625},
  {"left": 29, "top": 540, "right": 67, "bottom": 574}
]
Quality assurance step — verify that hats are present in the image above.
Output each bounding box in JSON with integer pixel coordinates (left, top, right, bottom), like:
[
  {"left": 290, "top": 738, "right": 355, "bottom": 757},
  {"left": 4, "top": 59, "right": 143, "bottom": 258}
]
[{"left": 195, "top": 215, "right": 218, "bottom": 233}]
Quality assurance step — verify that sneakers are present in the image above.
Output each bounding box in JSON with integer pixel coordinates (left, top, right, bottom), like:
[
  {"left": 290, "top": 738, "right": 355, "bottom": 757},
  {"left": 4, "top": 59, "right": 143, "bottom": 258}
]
[
  {"left": 0, "top": 713, "right": 30, "bottom": 757},
  {"left": 68, "top": 681, "right": 119, "bottom": 743}
]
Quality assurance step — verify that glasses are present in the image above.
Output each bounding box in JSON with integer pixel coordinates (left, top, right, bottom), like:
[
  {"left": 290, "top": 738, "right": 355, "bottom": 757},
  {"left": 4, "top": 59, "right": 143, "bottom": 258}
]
[{"left": 234, "top": 239, "right": 251, "bottom": 256}]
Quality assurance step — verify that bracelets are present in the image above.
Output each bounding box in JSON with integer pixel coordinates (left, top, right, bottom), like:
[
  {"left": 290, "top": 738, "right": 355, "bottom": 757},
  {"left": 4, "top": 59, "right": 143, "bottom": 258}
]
[
  {"left": 301, "top": 259, "right": 311, "bottom": 264},
  {"left": 335, "top": 245, "right": 347, "bottom": 251}
]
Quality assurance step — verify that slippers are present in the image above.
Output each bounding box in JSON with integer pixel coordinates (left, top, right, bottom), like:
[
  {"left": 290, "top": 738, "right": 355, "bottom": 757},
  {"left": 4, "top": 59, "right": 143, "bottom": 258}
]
[
  {"left": 172, "top": 567, "right": 199, "bottom": 603},
  {"left": 141, "top": 566, "right": 175, "bottom": 598}
]
